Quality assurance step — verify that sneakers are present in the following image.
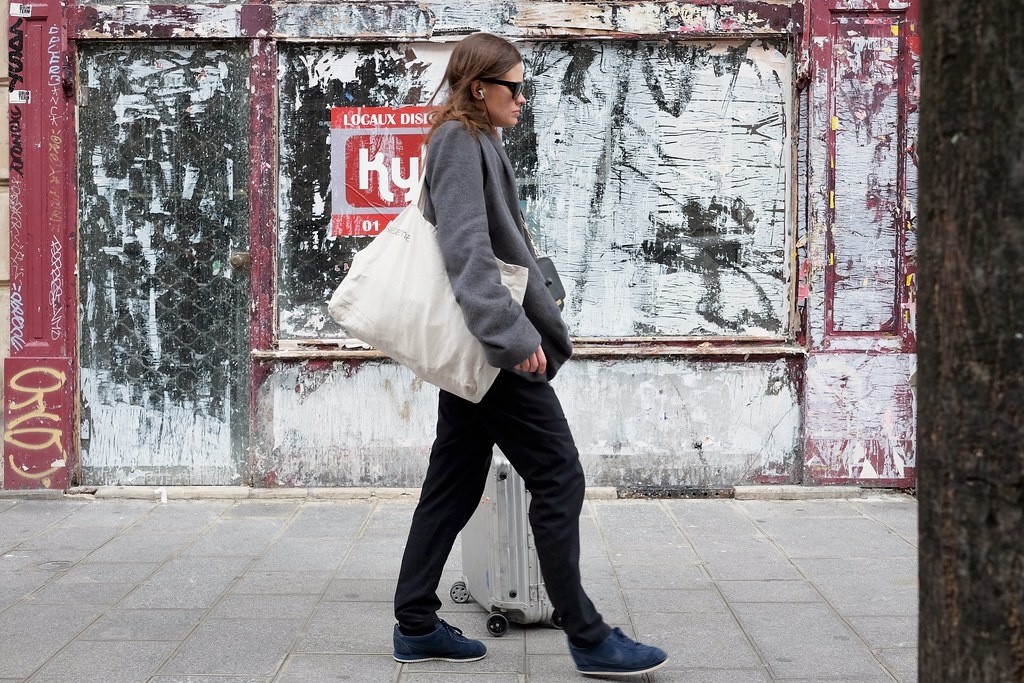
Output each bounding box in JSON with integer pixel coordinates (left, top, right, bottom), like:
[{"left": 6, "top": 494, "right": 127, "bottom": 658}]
[
  {"left": 392, "top": 618, "right": 487, "bottom": 663},
  {"left": 567, "top": 627, "right": 669, "bottom": 675}
]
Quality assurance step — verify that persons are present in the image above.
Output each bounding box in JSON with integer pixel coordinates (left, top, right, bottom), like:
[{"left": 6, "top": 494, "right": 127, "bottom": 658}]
[{"left": 391, "top": 33, "right": 670, "bottom": 676}]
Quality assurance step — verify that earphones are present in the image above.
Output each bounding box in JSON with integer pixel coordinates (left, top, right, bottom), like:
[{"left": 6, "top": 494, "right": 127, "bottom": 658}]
[{"left": 476, "top": 88, "right": 485, "bottom": 99}]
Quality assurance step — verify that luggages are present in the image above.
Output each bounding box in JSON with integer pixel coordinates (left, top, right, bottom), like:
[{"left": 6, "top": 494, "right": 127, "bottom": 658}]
[{"left": 450, "top": 453, "right": 567, "bottom": 637}]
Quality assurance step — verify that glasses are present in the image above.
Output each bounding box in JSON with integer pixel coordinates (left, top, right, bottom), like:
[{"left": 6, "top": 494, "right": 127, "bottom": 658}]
[{"left": 477, "top": 77, "right": 526, "bottom": 100}]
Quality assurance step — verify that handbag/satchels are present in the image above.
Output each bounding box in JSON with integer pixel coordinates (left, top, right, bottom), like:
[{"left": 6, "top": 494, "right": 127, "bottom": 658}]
[
  {"left": 535, "top": 257, "right": 566, "bottom": 314},
  {"left": 327, "top": 203, "right": 529, "bottom": 404}
]
